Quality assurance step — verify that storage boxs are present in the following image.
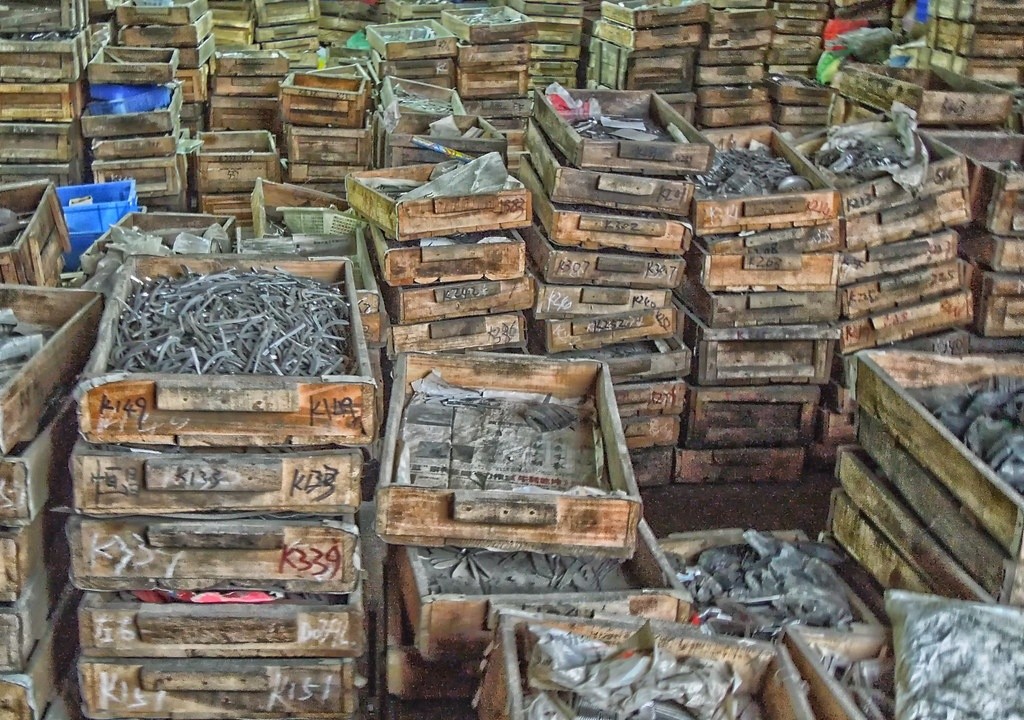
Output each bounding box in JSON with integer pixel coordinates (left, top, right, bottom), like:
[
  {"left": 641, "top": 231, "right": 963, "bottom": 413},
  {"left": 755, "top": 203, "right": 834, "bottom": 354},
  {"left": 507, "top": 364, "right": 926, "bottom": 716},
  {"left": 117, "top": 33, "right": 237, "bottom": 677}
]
[
  {"left": 90, "top": 83, "right": 150, "bottom": 100},
  {"left": 86, "top": 85, "right": 171, "bottom": 117},
  {"left": 55, "top": 179, "right": 147, "bottom": 274}
]
